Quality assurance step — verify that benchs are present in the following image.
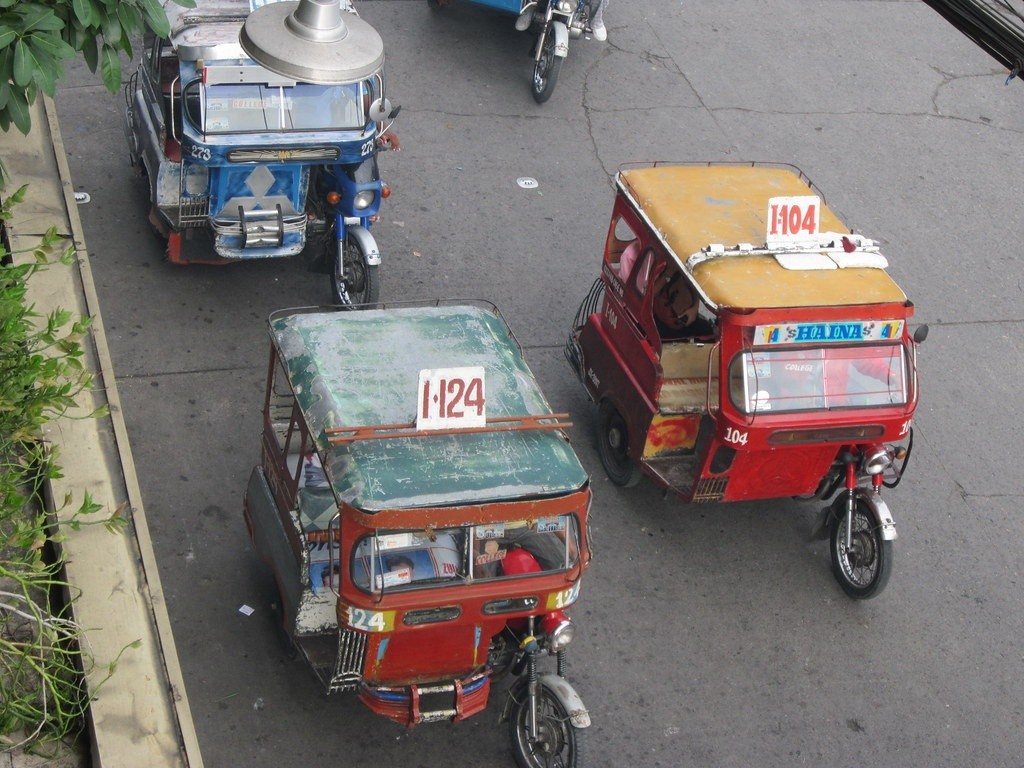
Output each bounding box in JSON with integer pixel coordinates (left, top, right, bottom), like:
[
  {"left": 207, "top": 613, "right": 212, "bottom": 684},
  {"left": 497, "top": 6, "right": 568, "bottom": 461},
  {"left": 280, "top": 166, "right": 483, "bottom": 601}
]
[
  {"left": 164, "top": 136, "right": 182, "bottom": 163},
  {"left": 302, "top": 530, "right": 465, "bottom": 594},
  {"left": 283, "top": 451, "right": 336, "bottom": 488},
  {"left": 658, "top": 342, "right": 772, "bottom": 414},
  {"left": 150, "top": 55, "right": 201, "bottom": 94}
]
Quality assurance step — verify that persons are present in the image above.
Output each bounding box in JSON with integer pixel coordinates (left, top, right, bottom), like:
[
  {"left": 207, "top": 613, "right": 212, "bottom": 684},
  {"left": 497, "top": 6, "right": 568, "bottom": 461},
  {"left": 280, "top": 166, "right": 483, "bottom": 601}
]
[
  {"left": 321, "top": 565, "right": 354, "bottom": 587},
  {"left": 473, "top": 520, "right": 579, "bottom": 579},
  {"left": 386, "top": 556, "right": 415, "bottom": 582},
  {"left": 616, "top": 234, "right": 719, "bottom": 344},
  {"left": 275, "top": 83, "right": 401, "bottom": 151},
  {"left": 769, "top": 346, "right": 903, "bottom": 410}
]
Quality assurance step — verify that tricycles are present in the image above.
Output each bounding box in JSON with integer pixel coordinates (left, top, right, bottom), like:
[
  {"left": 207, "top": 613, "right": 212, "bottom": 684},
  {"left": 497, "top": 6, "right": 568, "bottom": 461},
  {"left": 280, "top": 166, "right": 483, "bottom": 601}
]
[
  {"left": 241, "top": 294, "right": 593, "bottom": 768},
  {"left": 122, "top": 0, "right": 404, "bottom": 311},
  {"left": 422, "top": 1, "right": 609, "bottom": 103},
  {"left": 565, "top": 161, "right": 930, "bottom": 599}
]
